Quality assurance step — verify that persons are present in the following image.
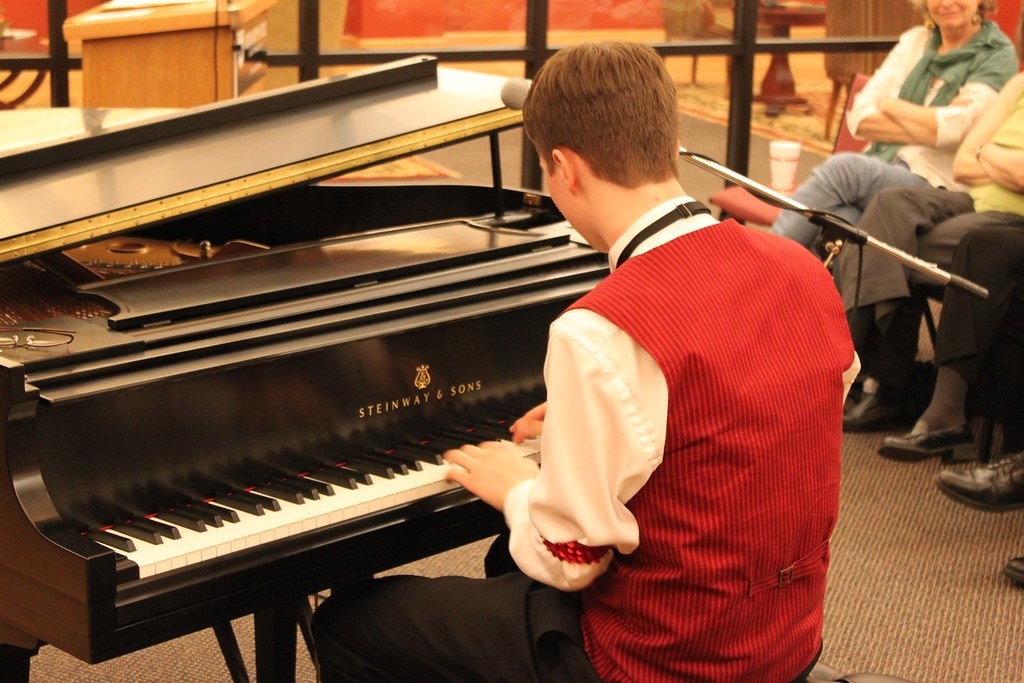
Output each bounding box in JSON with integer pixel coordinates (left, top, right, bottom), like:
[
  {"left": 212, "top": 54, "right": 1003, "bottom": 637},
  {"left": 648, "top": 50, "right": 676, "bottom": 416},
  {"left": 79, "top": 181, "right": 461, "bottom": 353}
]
[
  {"left": 770, "top": 0, "right": 1024, "bottom": 585},
  {"left": 311, "top": 44, "right": 862, "bottom": 683}
]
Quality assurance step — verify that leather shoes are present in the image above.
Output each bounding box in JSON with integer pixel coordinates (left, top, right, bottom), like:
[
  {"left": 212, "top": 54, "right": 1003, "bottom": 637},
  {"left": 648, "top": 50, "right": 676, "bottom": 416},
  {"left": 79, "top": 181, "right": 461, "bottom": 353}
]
[
  {"left": 1005, "top": 558, "right": 1024, "bottom": 584},
  {"left": 878, "top": 422, "right": 976, "bottom": 463},
  {"left": 936, "top": 450, "right": 1024, "bottom": 510}
]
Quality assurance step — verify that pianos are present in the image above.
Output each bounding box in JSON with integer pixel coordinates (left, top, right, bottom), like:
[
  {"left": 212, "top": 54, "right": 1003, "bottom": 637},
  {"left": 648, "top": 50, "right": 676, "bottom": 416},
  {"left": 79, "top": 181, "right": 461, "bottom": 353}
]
[{"left": 0, "top": 55, "right": 609, "bottom": 683}]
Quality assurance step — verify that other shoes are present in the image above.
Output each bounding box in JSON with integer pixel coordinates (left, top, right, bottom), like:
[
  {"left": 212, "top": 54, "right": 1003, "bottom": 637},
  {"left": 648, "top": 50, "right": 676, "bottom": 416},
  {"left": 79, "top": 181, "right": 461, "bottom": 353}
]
[
  {"left": 843, "top": 387, "right": 934, "bottom": 432},
  {"left": 849, "top": 383, "right": 864, "bottom": 405}
]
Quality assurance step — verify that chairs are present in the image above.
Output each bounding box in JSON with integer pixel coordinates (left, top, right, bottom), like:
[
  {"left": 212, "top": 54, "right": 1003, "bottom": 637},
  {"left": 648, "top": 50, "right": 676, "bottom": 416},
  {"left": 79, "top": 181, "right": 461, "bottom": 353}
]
[
  {"left": 652, "top": 0, "right": 737, "bottom": 102},
  {"left": 709, "top": 70, "right": 872, "bottom": 228}
]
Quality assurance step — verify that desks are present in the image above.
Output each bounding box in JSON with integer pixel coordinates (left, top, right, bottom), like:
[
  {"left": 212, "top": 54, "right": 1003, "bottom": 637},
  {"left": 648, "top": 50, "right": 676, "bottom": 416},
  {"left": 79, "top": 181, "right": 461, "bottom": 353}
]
[
  {"left": 744, "top": 5, "right": 827, "bottom": 116},
  {"left": 62, "top": 0, "right": 278, "bottom": 108}
]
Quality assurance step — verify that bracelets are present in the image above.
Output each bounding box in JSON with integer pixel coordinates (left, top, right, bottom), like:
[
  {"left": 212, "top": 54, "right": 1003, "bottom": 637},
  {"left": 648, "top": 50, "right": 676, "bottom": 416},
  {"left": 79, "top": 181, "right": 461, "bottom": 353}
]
[{"left": 975, "top": 142, "right": 992, "bottom": 160}]
